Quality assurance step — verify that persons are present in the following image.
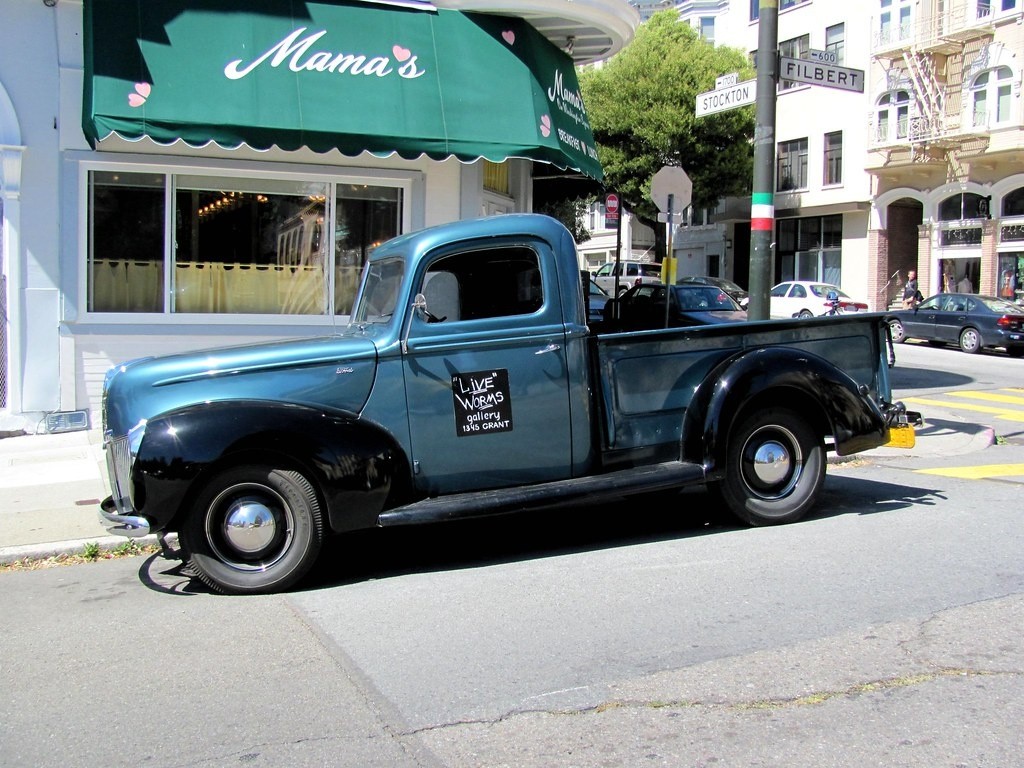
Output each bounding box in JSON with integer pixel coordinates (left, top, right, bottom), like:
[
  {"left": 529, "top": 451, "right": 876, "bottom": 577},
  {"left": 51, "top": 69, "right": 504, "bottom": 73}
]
[
  {"left": 902, "top": 271, "right": 917, "bottom": 309},
  {"left": 1001, "top": 270, "right": 1015, "bottom": 296},
  {"left": 957, "top": 278, "right": 974, "bottom": 294}
]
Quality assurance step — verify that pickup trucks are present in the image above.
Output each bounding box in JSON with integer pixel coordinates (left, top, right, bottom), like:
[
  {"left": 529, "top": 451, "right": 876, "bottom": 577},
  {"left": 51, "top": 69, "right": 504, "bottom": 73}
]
[{"left": 100, "top": 210, "right": 924, "bottom": 595}]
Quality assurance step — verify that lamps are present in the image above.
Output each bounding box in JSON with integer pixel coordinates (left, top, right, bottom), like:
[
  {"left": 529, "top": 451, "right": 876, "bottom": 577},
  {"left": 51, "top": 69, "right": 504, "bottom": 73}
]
[{"left": 562, "top": 37, "right": 576, "bottom": 53}]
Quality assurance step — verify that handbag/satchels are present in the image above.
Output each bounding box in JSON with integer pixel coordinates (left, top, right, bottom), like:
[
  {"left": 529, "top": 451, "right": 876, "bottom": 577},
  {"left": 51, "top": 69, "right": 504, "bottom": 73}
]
[{"left": 915, "top": 290, "right": 924, "bottom": 302}]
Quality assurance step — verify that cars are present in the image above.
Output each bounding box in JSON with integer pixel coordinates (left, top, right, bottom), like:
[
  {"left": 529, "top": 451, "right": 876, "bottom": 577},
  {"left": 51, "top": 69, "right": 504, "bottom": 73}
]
[
  {"left": 586, "top": 280, "right": 612, "bottom": 324},
  {"left": 602, "top": 284, "right": 750, "bottom": 334},
  {"left": 739, "top": 280, "right": 868, "bottom": 322},
  {"left": 674, "top": 277, "right": 749, "bottom": 312},
  {"left": 881, "top": 293, "right": 1024, "bottom": 354}
]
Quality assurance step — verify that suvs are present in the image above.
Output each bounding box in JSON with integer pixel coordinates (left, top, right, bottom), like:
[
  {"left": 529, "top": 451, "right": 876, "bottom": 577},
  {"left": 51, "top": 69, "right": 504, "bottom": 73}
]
[{"left": 591, "top": 260, "right": 663, "bottom": 300}]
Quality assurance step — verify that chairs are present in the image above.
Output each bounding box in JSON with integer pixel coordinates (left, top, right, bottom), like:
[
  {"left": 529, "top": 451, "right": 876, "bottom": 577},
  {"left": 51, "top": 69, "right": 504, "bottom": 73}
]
[{"left": 423, "top": 270, "right": 464, "bottom": 322}]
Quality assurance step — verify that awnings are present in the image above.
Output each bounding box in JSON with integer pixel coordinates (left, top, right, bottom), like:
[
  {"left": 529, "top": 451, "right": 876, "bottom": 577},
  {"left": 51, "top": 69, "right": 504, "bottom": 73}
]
[{"left": 84, "top": 0, "right": 607, "bottom": 187}]
